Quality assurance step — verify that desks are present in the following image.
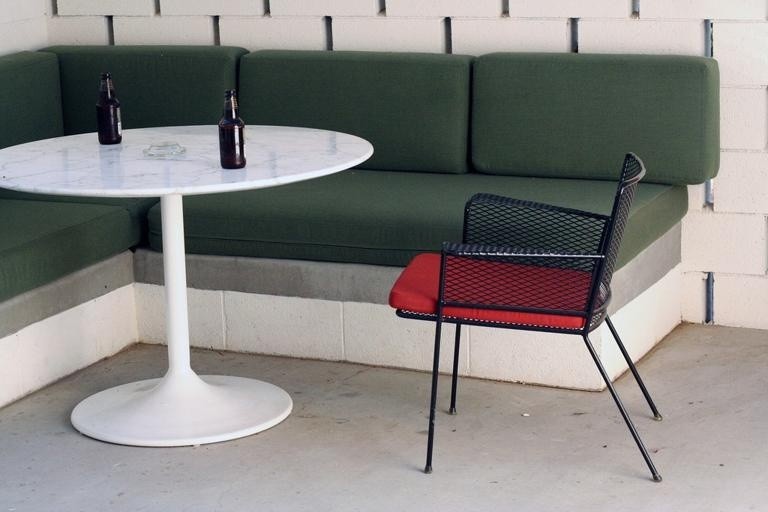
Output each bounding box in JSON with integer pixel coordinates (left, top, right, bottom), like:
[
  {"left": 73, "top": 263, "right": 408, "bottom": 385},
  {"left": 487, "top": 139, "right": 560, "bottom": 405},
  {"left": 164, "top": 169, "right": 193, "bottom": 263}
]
[{"left": 0, "top": 124, "right": 373, "bottom": 450}]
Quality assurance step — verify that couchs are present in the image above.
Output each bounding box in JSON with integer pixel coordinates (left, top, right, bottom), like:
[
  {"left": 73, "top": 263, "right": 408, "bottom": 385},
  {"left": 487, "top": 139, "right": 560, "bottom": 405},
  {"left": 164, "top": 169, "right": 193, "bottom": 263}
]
[{"left": 0, "top": 44, "right": 721, "bottom": 392}]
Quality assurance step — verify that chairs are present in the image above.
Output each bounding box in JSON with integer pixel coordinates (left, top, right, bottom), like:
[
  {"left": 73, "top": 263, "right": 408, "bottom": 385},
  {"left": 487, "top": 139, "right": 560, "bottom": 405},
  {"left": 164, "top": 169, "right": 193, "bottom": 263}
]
[{"left": 386, "top": 149, "right": 664, "bottom": 482}]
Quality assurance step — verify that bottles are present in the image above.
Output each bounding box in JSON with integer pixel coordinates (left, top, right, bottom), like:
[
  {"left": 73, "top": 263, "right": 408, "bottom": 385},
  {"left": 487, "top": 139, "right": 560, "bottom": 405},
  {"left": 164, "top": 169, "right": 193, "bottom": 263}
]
[
  {"left": 218, "top": 88, "right": 248, "bottom": 169},
  {"left": 95, "top": 72, "right": 123, "bottom": 145}
]
